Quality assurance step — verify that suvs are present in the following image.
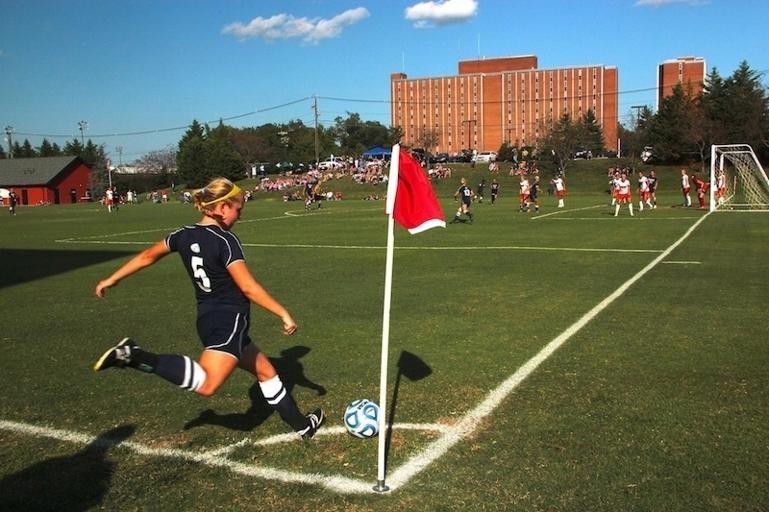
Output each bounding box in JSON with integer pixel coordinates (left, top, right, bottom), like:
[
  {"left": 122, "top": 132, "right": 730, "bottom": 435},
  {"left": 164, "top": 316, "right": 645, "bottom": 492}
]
[{"left": 319, "top": 157, "right": 345, "bottom": 170}]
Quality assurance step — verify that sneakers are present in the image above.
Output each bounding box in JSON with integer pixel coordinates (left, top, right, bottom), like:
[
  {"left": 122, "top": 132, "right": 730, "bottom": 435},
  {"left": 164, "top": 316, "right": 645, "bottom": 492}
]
[
  {"left": 298, "top": 409, "right": 324, "bottom": 441},
  {"left": 94, "top": 338, "right": 142, "bottom": 372}
]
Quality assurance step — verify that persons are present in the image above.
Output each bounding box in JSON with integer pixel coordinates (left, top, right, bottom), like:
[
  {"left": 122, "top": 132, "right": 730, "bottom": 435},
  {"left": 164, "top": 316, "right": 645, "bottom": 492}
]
[
  {"left": 642, "top": 155, "right": 648, "bottom": 164},
  {"left": 680, "top": 168, "right": 725, "bottom": 210},
  {"left": 8, "top": 189, "right": 17, "bottom": 216},
  {"left": 85, "top": 189, "right": 92, "bottom": 198},
  {"left": 69, "top": 184, "right": 76, "bottom": 203},
  {"left": 243, "top": 154, "right": 391, "bottom": 213},
  {"left": 571, "top": 152, "right": 604, "bottom": 160},
  {"left": 412, "top": 152, "right": 567, "bottom": 225},
  {"left": 95, "top": 177, "right": 326, "bottom": 442},
  {"left": 606, "top": 164, "right": 659, "bottom": 216},
  {"left": 102, "top": 184, "right": 193, "bottom": 213},
  {"left": 0, "top": 196, "right": 4, "bottom": 207}
]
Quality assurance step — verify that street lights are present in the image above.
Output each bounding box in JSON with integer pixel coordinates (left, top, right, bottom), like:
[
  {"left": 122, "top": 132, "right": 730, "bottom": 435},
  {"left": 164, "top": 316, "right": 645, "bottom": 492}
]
[
  {"left": 5, "top": 125, "right": 16, "bottom": 150},
  {"left": 78, "top": 120, "right": 90, "bottom": 148},
  {"left": 115, "top": 144, "right": 123, "bottom": 165}
]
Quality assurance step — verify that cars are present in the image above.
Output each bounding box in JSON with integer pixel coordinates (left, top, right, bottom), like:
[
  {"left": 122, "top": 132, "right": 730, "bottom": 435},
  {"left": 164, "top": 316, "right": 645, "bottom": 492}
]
[
  {"left": 432, "top": 153, "right": 449, "bottom": 162},
  {"left": 476, "top": 151, "right": 498, "bottom": 162},
  {"left": 450, "top": 156, "right": 470, "bottom": 163}
]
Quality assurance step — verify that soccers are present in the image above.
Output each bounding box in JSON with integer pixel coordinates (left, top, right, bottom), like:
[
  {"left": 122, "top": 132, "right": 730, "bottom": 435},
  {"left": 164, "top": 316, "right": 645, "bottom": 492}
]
[{"left": 344, "top": 399, "right": 378, "bottom": 438}]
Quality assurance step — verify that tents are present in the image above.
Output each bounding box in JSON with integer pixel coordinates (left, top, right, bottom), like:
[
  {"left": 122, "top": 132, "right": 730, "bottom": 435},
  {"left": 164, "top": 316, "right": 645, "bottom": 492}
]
[{"left": 362, "top": 147, "right": 392, "bottom": 160}]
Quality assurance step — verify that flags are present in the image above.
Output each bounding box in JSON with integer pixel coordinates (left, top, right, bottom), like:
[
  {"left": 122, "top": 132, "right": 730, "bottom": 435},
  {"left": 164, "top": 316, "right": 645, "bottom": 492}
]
[{"left": 394, "top": 147, "right": 446, "bottom": 235}]
